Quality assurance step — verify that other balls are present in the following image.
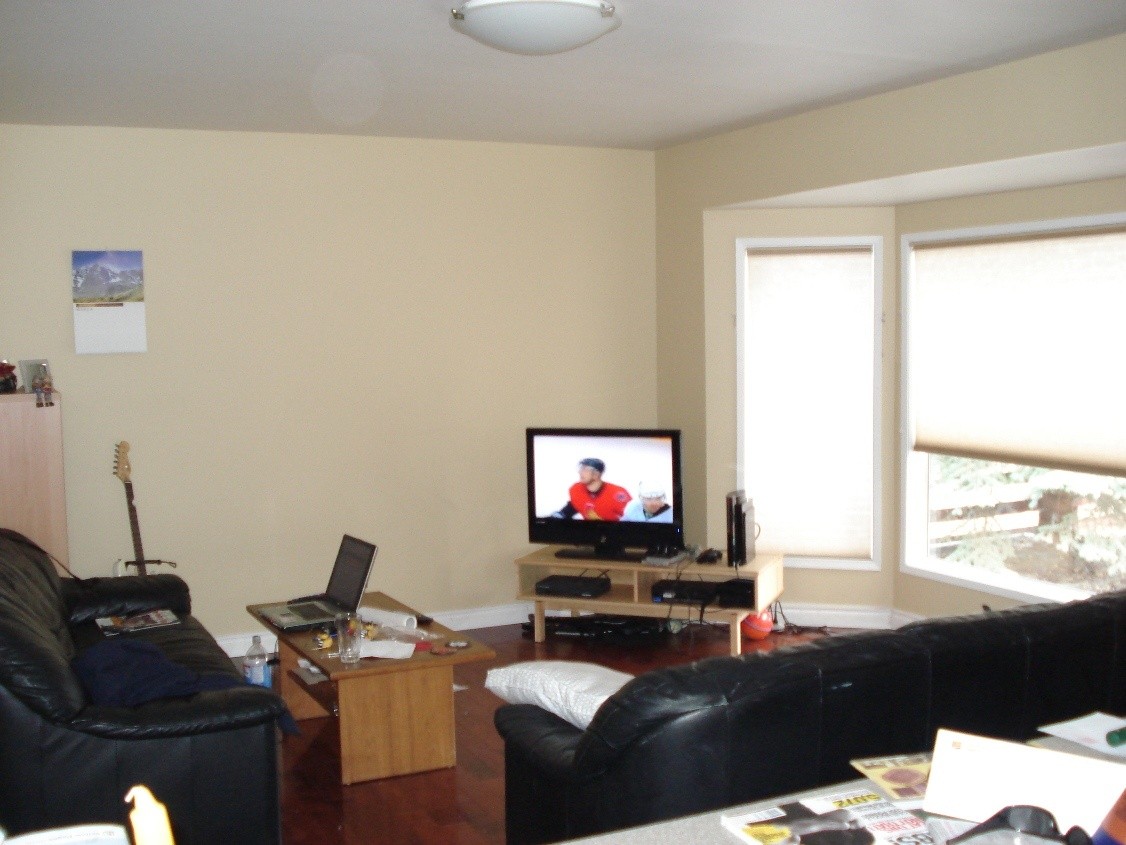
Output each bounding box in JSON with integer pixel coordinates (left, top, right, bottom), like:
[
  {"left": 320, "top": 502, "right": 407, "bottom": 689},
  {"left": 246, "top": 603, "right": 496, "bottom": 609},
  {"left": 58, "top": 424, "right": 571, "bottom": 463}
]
[{"left": 741, "top": 608, "right": 772, "bottom": 642}]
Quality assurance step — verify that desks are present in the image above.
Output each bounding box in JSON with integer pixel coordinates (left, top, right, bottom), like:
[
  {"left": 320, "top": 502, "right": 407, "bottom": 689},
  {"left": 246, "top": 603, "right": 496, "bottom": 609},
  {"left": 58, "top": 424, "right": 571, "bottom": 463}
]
[{"left": 553, "top": 720, "right": 1126, "bottom": 845}]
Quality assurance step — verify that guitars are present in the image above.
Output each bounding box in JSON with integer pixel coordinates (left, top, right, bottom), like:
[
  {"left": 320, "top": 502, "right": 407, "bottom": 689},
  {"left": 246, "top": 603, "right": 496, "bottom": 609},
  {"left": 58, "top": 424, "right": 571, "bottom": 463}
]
[{"left": 112, "top": 440, "right": 172, "bottom": 577}]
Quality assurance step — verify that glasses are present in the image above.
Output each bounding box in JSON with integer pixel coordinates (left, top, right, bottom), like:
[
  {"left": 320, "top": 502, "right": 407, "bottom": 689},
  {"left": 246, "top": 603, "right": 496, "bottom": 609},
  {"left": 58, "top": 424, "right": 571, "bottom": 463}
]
[{"left": 945, "top": 803, "right": 1096, "bottom": 845}]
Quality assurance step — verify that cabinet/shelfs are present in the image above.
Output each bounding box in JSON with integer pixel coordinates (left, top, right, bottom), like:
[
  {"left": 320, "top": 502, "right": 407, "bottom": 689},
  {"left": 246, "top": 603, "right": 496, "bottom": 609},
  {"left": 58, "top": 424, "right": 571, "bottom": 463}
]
[
  {"left": 0, "top": 387, "right": 71, "bottom": 577},
  {"left": 514, "top": 545, "right": 783, "bottom": 658}
]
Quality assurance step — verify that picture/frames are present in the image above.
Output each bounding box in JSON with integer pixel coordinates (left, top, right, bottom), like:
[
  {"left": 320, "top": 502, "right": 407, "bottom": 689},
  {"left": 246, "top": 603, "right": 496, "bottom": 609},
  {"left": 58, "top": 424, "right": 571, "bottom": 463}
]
[{"left": 18, "top": 359, "right": 54, "bottom": 393}]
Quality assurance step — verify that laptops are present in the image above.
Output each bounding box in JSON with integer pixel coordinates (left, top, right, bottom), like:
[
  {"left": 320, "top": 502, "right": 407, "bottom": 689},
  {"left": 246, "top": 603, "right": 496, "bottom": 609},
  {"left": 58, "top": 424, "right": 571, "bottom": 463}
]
[{"left": 258, "top": 534, "right": 377, "bottom": 632}]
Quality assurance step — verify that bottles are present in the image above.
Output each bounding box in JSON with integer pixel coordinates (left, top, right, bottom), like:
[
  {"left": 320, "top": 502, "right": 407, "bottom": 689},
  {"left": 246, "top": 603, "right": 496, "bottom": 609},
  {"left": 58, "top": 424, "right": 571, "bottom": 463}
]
[{"left": 243, "top": 636, "right": 272, "bottom": 689}]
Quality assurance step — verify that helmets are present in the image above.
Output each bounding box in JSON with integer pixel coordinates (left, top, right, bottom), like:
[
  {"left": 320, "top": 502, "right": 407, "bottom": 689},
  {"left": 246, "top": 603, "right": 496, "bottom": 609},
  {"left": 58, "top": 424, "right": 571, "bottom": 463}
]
[{"left": 638, "top": 480, "right": 667, "bottom": 498}]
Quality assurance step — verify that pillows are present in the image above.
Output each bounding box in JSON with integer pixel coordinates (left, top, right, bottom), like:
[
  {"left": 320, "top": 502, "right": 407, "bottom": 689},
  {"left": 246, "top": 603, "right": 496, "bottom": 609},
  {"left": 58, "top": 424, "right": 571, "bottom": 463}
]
[{"left": 484, "top": 654, "right": 641, "bottom": 729}]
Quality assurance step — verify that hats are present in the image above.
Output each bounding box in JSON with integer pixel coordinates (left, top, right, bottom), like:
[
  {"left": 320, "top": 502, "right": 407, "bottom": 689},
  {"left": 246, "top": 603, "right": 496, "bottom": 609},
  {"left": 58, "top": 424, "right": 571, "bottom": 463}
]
[{"left": 580, "top": 458, "right": 606, "bottom": 472}]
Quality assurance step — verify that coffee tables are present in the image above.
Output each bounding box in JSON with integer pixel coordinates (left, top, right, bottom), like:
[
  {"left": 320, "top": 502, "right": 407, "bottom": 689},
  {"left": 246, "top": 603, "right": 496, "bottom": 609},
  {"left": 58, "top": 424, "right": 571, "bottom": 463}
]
[{"left": 272, "top": 590, "right": 504, "bottom": 787}]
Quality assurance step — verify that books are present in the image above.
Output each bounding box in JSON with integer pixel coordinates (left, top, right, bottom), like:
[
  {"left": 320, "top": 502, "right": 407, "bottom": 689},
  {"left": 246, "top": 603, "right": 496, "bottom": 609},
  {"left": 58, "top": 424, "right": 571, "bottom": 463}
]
[
  {"left": 721, "top": 751, "right": 931, "bottom": 845},
  {"left": 95, "top": 609, "right": 181, "bottom": 636}
]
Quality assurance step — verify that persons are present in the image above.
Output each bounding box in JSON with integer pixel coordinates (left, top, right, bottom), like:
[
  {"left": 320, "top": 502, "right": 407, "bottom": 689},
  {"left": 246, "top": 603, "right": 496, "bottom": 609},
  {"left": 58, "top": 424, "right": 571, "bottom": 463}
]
[{"left": 544, "top": 459, "right": 672, "bottom": 522}]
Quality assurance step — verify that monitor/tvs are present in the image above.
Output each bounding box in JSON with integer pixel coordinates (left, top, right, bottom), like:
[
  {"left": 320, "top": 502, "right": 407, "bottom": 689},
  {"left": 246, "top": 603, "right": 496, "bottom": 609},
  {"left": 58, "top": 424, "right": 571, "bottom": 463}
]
[{"left": 527, "top": 428, "right": 682, "bottom": 563}]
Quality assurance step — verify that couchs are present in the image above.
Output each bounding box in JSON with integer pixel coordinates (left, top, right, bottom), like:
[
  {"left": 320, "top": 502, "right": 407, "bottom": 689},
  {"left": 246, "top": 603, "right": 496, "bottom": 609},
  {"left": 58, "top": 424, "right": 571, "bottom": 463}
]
[
  {"left": 0, "top": 527, "right": 303, "bottom": 845},
  {"left": 493, "top": 582, "right": 1126, "bottom": 845}
]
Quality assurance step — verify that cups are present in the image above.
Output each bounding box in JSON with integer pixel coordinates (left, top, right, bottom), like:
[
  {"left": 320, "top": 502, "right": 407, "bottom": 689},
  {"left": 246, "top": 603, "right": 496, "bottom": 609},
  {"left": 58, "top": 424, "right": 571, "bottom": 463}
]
[{"left": 335, "top": 613, "right": 362, "bottom": 664}]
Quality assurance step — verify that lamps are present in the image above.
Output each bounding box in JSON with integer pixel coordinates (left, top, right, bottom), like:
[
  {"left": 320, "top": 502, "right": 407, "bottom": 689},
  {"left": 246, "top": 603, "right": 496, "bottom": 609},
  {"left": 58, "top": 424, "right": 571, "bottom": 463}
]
[{"left": 447, "top": 0, "right": 623, "bottom": 55}]
